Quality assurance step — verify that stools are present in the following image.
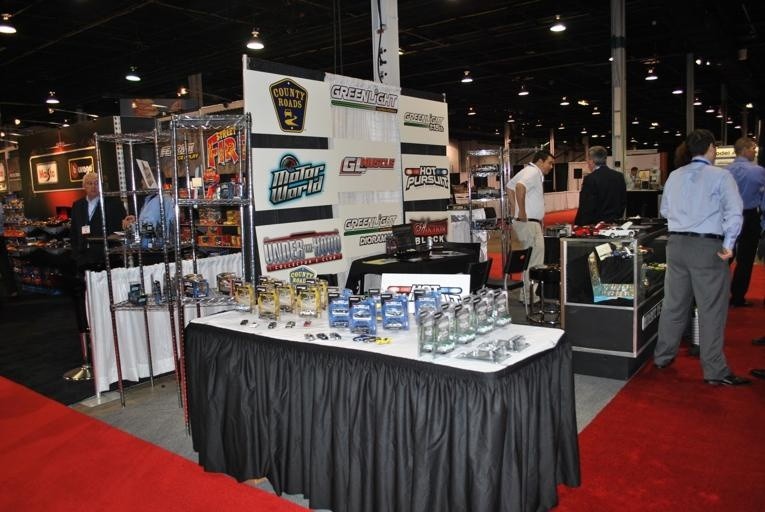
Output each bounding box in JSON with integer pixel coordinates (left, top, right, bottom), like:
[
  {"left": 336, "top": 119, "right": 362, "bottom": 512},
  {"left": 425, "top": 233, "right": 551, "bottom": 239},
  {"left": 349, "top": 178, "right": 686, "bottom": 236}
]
[{"left": 525, "top": 263, "right": 561, "bottom": 328}]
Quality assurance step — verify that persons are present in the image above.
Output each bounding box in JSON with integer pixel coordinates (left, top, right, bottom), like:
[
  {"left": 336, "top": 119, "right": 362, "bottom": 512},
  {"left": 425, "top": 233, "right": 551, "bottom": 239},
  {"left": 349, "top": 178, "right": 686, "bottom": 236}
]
[
  {"left": 505, "top": 150, "right": 556, "bottom": 306},
  {"left": 119, "top": 167, "right": 181, "bottom": 252},
  {"left": 649, "top": 128, "right": 755, "bottom": 387},
  {"left": 68, "top": 170, "right": 128, "bottom": 242},
  {"left": 725, "top": 136, "right": 765, "bottom": 308},
  {"left": 573, "top": 145, "right": 630, "bottom": 228},
  {"left": 626, "top": 166, "right": 640, "bottom": 191}
]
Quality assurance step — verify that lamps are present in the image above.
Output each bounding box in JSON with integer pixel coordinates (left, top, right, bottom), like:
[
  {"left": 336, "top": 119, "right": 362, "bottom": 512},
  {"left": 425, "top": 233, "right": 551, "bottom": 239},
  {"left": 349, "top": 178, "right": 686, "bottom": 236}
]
[
  {"left": 559, "top": 97, "right": 569, "bottom": 106},
  {"left": 592, "top": 122, "right": 685, "bottom": 146},
  {"left": 717, "top": 109, "right": 723, "bottom": 118},
  {"left": 632, "top": 118, "right": 639, "bottom": 124},
  {"left": 746, "top": 101, "right": 754, "bottom": 108},
  {"left": 461, "top": 71, "right": 474, "bottom": 83},
  {"left": 245, "top": 26, "right": 265, "bottom": 50},
  {"left": 549, "top": 14, "right": 566, "bottom": 33},
  {"left": 727, "top": 115, "right": 734, "bottom": 123},
  {"left": 124, "top": 64, "right": 142, "bottom": 82},
  {"left": 507, "top": 114, "right": 514, "bottom": 123},
  {"left": 706, "top": 104, "right": 713, "bottom": 114},
  {"left": 691, "top": 58, "right": 725, "bottom": 68},
  {"left": 693, "top": 97, "right": 702, "bottom": 106},
  {"left": 592, "top": 107, "right": 600, "bottom": 116},
  {"left": 518, "top": 83, "right": 529, "bottom": 97},
  {"left": 0, "top": 8, "right": 17, "bottom": 35},
  {"left": 716, "top": 125, "right": 764, "bottom": 149},
  {"left": 645, "top": 68, "right": 657, "bottom": 81},
  {"left": 535, "top": 120, "right": 542, "bottom": 127},
  {"left": 559, "top": 124, "right": 565, "bottom": 129},
  {"left": 467, "top": 106, "right": 475, "bottom": 116},
  {"left": 672, "top": 83, "right": 685, "bottom": 95},
  {"left": 45, "top": 89, "right": 60, "bottom": 105},
  {"left": 580, "top": 128, "right": 587, "bottom": 134}
]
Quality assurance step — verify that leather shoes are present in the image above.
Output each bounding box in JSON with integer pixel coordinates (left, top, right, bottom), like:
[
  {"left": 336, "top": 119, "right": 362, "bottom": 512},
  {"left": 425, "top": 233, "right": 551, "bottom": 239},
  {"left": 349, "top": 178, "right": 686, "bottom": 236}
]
[
  {"left": 750, "top": 369, "right": 765, "bottom": 378},
  {"left": 704, "top": 373, "right": 752, "bottom": 386},
  {"left": 752, "top": 336, "right": 765, "bottom": 345},
  {"left": 741, "top": 301, "right": 753, "bottom": 307}
]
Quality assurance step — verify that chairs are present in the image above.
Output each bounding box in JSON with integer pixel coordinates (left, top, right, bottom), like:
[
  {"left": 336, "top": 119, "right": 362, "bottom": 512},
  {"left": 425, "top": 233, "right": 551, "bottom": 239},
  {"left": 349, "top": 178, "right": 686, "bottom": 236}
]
[
  {"left": 466, "top": 257, "right": 493, "bottom": 294},
  {"left": 487, "top": 245, "right": 533, "bottom": 317}
]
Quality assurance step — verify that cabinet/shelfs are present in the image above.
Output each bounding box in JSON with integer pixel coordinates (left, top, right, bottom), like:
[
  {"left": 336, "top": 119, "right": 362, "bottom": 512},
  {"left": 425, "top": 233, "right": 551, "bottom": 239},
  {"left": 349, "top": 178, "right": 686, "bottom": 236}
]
[
  {"left": 558, "top": 220, "right": 671, "bottom": 380},
  {"left": 625, "top": 188, "right": 664, "bottom": 219},
  {"left": 169, "top": 111, "right": 259, "bottom": 436},
  {"left": 463, "top": 145, "right": 515, "bottom": 287},
  {"left": 93, "top": 128, "right": 183, "bottom": 408},
  {"left": 0, "top": 180, "right": 244, "bottom": 296}
]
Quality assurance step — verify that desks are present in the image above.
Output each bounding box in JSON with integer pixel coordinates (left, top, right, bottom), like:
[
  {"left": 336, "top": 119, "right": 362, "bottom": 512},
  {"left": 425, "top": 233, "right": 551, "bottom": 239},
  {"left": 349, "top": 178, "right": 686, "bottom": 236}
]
[
  {"left": 346, "top": 243, "right": 480, "bottom": 296},
  {"left": 183, "top": 292, "right": 583, "bottom": 510}
]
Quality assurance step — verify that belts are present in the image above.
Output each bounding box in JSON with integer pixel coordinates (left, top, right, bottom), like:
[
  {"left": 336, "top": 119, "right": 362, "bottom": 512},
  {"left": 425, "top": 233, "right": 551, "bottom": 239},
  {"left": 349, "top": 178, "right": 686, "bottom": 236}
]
[
  {"left": 514, "top": 217, "right": 542, "bottom": 223},
  {"left": 670, "top": 231, "right": 724, "bottom": 238}
]
